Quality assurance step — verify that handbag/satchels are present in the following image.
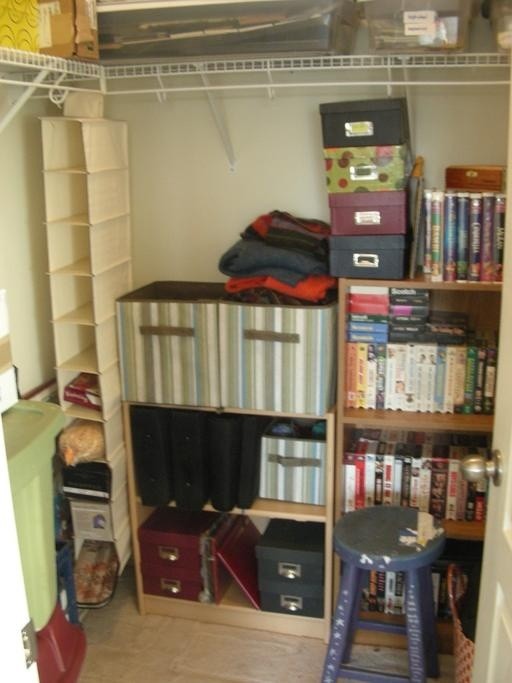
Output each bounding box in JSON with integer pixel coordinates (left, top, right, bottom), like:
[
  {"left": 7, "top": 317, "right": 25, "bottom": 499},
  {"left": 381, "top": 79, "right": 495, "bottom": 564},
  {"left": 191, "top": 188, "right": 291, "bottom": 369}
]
[{"left": 446, "top": 562, "right": 482, "bottom": 683}]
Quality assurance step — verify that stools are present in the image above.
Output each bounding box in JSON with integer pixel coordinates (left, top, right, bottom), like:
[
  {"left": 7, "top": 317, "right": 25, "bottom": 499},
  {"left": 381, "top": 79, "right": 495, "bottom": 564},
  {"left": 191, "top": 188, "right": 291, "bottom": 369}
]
[{"left": 320, "top": 503, "right": 446, "bottom": 683}]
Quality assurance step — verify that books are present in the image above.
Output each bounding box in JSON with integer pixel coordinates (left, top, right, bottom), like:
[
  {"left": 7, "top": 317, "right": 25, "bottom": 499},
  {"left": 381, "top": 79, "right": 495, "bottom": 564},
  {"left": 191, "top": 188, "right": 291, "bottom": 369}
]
[
  {"left": 353, "top": 550, "right": 456, "bottom": 625},
  {"left": 342, "top": 427, "right": 488, "bottom": 527},
  {"left": 422, "top": 189, "right": 506, "bottom": 284},
  {"left": 346, "top": 286, "right": 499, "bottom": 417}
]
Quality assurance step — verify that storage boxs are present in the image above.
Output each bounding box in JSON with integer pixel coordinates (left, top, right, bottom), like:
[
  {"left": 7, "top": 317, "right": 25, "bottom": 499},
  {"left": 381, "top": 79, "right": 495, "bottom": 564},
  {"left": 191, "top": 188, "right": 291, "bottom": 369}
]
[
  {"left": 138, "top": 509, "right": 223, "bottom": 562},
  {"left": 261, "top": 585, "right": 322, "bottom": 621},
  {"left": 259, "top": 520, "right": 325, "bottom": 583},
  {"left": 363, "top": 0, "right": 475, "bottom": 54},
  {"left": 256, "top": 434, "right": 325, "bottom": 509},
  {"left": 484, "top": 1, "right": 512, "bottom": 61},
  {"left": 222, "top": 302, "right": 338, "bottom": 412},
  {"left": 320, "top": 96, "right": 410, "bottom": 147},
  {"left": 330, "top": 237, "right": 404, "bottom": 278},
  {"left": 116, "top": 299, "right": 220, "bottom": 409},
  {"left": 1, "top": 0, "right": 39, "bottom": 54},
  {"left": 41, "top": 1, "right": 99, "bottom": 56},
  {"left": 100, "top": 1, "right": 353, "bottom": 63},
  {"left": 142, "top": 565, "right": 216, "bottom": 603},
  {"left": 329, "top": 194, "right": 407, "bottom": 234},
  {"left": 324, "top": 150, "right": 407, "bottom": 191}
]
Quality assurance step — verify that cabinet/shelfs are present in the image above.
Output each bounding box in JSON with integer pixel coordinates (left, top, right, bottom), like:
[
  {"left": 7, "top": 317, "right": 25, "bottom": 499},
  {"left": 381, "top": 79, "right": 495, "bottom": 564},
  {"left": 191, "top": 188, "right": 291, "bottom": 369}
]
[
  {"left": 41, "top": 117, "right": 132, "bottom": 582},
  {"left": 325, "top": 276, "right": 499, "bottom": 647}
]
